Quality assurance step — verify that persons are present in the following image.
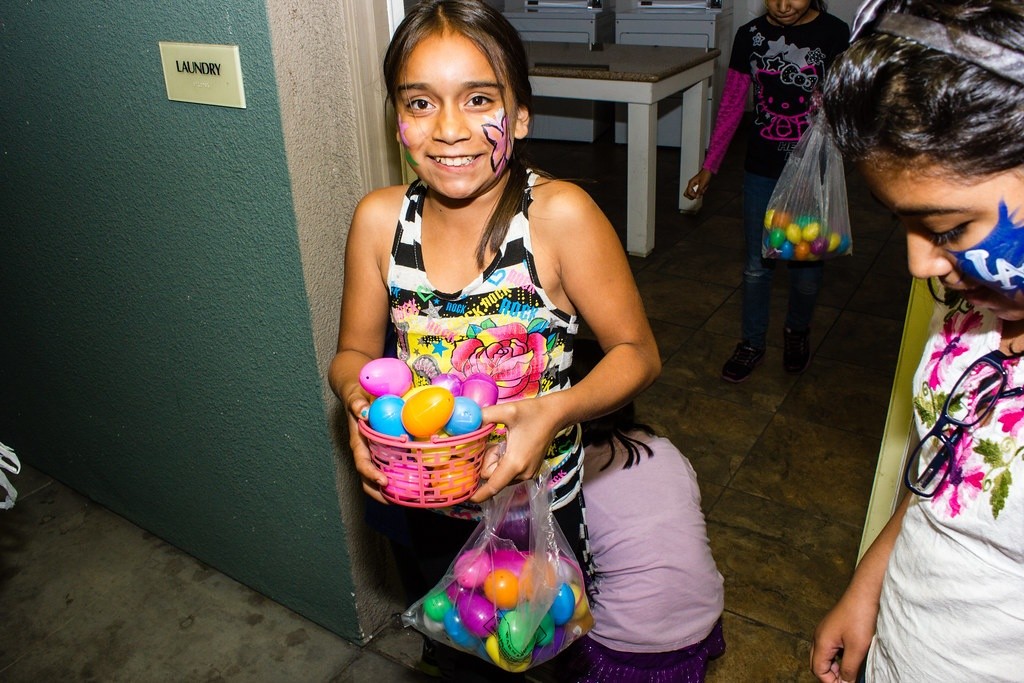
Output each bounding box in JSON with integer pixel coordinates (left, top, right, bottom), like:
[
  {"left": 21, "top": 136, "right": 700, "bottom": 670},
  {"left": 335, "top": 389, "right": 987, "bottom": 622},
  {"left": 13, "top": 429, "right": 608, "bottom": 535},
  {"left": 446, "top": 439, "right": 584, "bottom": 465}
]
[
  {"left": 683, "top": 0, "right": 849, "bottom": 386},
  {"left": 327, "top": 1, "right": 664, "bottom": 683},
  {"left": 812, "top": 1, "right": 1024, "bottom": 683},
  {"left": 563, "top": 338, "right": 727, "bottom": 683}
]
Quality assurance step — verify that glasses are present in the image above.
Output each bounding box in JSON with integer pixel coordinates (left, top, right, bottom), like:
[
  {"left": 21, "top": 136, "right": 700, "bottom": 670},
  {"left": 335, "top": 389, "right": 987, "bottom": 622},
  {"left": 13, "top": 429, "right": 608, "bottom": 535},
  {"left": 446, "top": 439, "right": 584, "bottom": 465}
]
[{"left": 904, "top": 349, "right": 1024, "bottom": 498}]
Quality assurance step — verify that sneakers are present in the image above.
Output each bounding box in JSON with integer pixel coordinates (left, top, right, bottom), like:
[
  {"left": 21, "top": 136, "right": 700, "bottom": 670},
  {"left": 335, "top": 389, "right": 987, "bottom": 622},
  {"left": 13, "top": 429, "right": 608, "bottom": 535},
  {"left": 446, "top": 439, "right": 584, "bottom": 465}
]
[
  {"left": 720, "top": 340, "right": 766, "bottom": 382},
  {"left": 782, "top": 328, "right": 810, "bottom": 375}
]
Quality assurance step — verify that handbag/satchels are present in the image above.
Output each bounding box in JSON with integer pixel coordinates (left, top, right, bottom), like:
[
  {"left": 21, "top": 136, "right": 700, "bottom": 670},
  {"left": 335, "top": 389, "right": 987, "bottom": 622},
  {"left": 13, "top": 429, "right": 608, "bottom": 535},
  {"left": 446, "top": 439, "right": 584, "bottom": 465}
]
[{"left": 761, "top": 106, "right": 853, "bottom": 263}]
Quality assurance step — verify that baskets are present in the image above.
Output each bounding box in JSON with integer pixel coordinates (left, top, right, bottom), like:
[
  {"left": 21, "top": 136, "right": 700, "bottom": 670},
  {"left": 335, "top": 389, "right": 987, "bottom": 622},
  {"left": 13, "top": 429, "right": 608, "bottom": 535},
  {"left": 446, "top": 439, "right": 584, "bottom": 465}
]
[{"left": 357, "top": 412, "right": 495, "bottom": 509}]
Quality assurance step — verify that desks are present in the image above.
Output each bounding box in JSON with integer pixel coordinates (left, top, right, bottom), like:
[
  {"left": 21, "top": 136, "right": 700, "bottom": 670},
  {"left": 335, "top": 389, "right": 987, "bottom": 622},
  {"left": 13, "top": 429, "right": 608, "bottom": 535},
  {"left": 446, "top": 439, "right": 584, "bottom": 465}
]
[{"left": 520, "top": 40, "right": 722, "bottom": 258}]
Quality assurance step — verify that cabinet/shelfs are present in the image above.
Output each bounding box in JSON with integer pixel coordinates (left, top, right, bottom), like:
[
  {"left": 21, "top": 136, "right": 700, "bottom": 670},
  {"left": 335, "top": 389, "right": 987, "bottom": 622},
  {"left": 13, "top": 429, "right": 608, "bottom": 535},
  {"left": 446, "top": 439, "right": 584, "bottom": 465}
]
[
  {"left": 615, "top": 6, "right": 734, "bottom": 150},
  {"left": 500, "top": 6, "right": 616, "bottom": 142}
]
